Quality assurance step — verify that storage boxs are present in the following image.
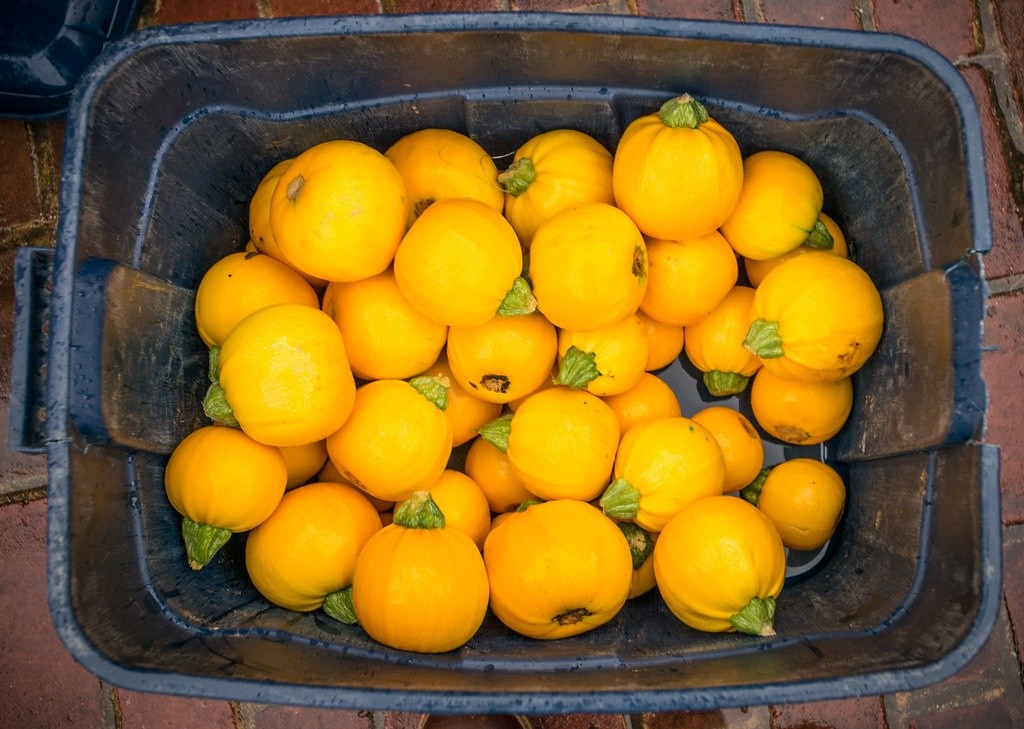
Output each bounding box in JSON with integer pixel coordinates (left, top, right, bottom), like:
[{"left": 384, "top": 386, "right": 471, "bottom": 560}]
[{"left": 8, "top": 9, "right": 1003, "bottom": 713}]
